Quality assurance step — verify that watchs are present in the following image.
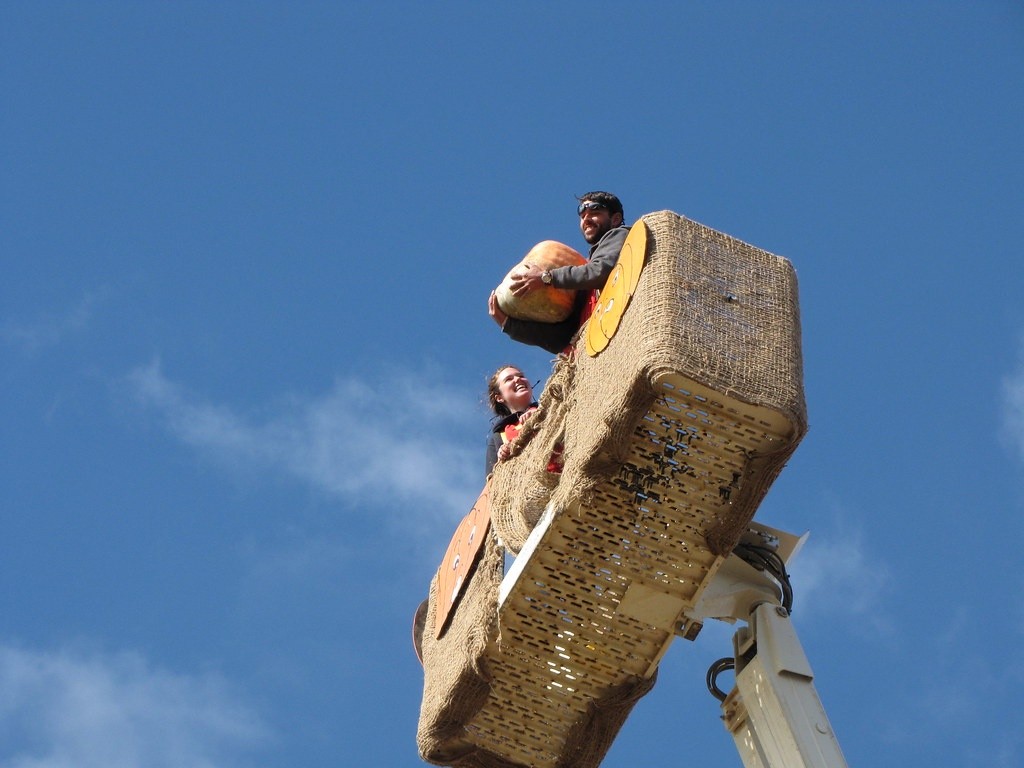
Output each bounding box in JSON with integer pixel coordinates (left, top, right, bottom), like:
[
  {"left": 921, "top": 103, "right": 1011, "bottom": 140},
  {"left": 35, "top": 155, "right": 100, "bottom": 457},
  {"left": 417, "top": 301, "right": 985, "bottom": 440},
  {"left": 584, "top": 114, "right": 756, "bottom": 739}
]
[{"left": 540, "top": 267, "right": 553, "bottom": 287}]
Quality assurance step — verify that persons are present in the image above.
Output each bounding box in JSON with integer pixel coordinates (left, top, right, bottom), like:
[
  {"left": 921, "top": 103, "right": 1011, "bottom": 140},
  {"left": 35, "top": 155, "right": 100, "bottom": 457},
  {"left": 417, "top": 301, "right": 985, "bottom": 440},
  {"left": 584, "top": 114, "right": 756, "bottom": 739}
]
[
  {"left": 487, "top": 190, "right": 633, "bottom": 355},
  {"left": 484, "top": 364, "right": 540, "bottom": 482}
]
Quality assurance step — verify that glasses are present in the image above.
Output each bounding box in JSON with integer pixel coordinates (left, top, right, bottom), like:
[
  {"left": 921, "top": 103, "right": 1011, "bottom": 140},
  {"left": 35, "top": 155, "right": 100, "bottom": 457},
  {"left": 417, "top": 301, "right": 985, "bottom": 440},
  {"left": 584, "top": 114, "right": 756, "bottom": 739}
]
[{"left": 578, "top": 201, "right": 609, "bottom": 216}]
